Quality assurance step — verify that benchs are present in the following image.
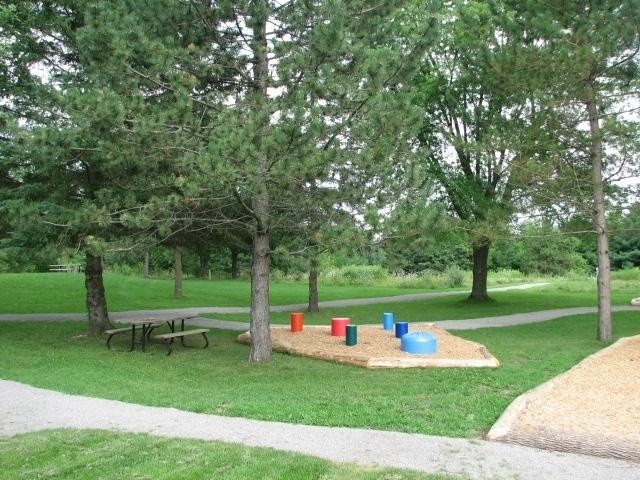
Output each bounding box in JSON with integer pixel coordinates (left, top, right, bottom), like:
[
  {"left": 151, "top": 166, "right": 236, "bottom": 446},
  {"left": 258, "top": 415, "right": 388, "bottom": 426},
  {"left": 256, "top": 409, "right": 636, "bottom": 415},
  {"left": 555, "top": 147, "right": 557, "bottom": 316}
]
[
  {"left": 154, "top": 329, "right": 212, "bottom": 356},
  {"left": 104, "top": 323, "right": 163, "bottom": 349}
]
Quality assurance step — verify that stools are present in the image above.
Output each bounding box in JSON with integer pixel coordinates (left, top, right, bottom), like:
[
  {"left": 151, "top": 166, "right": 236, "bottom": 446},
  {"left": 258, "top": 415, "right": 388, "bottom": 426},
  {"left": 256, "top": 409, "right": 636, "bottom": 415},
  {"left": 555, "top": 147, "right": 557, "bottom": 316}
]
[
  {"left": 395, "top": 322, "right": 408, "bottom": 337},
  {"left": 345, "top": 325, "right": 357, "bottom": 346},
  {"left": 332, "top": 318, "right": 351, "bottom": 337},
  {"left": 291, "top": 313, "right": 304, "bottom": 332},
  {"left": 383, "top": 312, "right": 393, "bottom": 329},
  {"left": 400, "top": 331, "right": 436, "bottom": 354}
]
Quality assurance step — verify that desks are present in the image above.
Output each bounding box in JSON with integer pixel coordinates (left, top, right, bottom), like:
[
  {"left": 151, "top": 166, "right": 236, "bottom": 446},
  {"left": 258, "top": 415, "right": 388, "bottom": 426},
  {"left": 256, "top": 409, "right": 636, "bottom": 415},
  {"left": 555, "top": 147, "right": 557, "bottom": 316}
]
[{"left": 112, "top": 314, "right": 203, "bottom": 351}]
